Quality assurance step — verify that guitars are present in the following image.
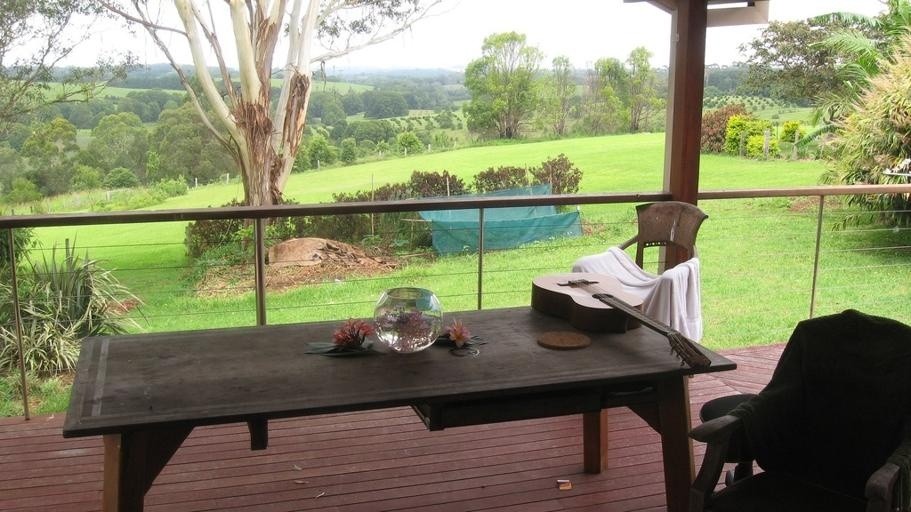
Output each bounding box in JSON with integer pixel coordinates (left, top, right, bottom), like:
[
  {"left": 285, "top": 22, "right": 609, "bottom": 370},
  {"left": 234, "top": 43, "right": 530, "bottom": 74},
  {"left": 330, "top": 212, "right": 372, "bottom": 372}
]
[{"left": 532, "top": 271, "right": 712, "bottom": 370}]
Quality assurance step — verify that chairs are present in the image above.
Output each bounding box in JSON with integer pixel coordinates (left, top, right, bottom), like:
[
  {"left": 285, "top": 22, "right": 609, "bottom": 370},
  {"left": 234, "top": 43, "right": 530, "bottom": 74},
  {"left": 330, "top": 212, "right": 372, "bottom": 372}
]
[
  {"left": 686, "top": 309, "right": 911, "bottom": 509},
  {"left": 616, "top": 199, "right": 706, "bottom": 270}
]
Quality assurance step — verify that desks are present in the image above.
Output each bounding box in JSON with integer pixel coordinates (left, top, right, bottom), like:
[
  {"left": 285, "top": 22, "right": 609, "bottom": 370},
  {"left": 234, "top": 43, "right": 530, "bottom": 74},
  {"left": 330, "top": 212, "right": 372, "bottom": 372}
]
[{"left": 62, "top": 302, "right": 737, "bottom": 511}]
